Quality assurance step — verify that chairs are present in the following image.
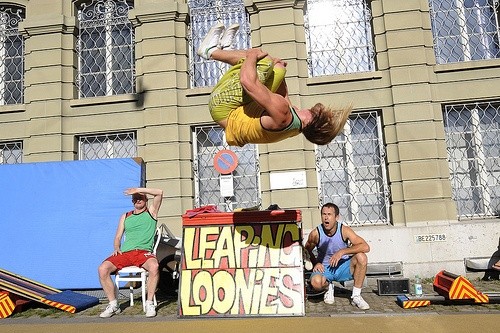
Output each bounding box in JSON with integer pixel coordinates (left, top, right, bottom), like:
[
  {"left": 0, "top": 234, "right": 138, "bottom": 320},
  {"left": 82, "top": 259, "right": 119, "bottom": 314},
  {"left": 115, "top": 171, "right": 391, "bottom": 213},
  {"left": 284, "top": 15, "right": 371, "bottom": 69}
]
[{"left": 114, "top": 226, "right": 162, "bottom": 313}]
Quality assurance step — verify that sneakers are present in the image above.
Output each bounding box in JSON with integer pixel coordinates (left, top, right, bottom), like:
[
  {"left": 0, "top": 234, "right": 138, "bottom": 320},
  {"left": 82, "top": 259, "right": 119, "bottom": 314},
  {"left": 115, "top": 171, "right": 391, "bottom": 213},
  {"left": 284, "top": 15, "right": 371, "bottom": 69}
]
[
  {"left": 351, "top": 295, "right": 370, "bottom": 310},
  {"left": 145, "top": 305, "right": 157, "bottom": 318},
  {"left": 221, "top": 24, "right": 240, "bottom": 49},
  {"left": 197, "top": 24, "right": 224, "bottom": 59},
  {"left": 100, "top": 303, "right": 121, "bottom": 318},
  {"left": 324, "top": 283, "right": 334, "bottom": 304}
]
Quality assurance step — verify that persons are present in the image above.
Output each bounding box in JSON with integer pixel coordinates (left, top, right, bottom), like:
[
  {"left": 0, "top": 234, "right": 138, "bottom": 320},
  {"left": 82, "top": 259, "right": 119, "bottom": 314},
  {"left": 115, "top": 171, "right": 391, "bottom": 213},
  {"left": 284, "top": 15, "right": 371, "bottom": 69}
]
[
  {"left": 304, "top": 202, "right": 371, "bottom": 309},
  {"left": 98, "top": 188, "right": 163, "bottom": 318},
  {"left": 197, "top": 23, "right": 352, "bottom": 147}
]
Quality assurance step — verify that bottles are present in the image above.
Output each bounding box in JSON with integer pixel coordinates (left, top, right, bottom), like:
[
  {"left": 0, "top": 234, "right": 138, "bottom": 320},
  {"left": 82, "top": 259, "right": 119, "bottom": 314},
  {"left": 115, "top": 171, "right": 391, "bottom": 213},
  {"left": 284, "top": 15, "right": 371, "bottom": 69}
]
[{"left": 414, "top": 275, "right": 422, "bottom": 295}]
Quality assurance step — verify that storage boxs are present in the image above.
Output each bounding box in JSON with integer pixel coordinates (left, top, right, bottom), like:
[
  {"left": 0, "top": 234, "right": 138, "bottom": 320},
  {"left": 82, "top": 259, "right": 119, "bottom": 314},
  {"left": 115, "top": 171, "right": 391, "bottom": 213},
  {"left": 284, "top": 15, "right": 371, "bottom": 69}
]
[{"left": 376, "top": 277, "right": 411, "bottom": 296}]
